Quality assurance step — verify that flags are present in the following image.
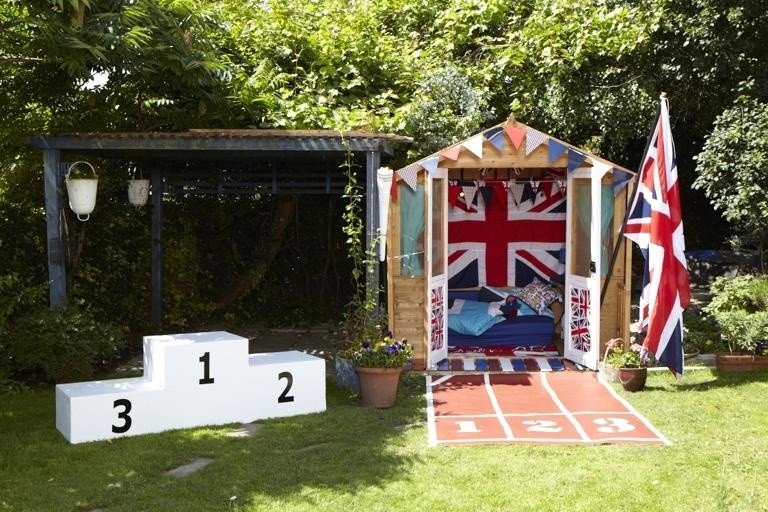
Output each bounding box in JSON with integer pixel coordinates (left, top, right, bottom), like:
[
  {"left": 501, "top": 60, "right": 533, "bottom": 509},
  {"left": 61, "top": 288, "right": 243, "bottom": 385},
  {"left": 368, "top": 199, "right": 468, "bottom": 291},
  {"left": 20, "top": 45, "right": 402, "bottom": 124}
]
[{"left": 625, "top": 98, "right": 704, "bottom": 385}]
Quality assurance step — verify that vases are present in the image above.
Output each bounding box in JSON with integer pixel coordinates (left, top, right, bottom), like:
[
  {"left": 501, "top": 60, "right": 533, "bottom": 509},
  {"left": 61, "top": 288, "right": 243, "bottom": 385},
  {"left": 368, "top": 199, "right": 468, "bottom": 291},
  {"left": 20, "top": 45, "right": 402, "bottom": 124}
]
[{"left": 355, "top": 367, "right": 402, "bottom": 408}]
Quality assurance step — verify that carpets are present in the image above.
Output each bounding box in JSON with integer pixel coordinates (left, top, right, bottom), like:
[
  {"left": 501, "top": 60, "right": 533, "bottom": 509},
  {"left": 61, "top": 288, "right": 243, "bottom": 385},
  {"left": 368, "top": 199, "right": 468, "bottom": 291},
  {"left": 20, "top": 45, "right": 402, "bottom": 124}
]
[{"left": 424, "top": 370, "right": 670, "bottom": 449}]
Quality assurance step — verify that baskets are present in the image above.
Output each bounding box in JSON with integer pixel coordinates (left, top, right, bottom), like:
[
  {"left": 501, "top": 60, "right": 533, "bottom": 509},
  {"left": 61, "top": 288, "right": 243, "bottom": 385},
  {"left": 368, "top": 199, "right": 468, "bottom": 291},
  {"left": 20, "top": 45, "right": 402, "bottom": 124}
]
[{"left": 597, "top": 337, "right": 626, "bottom": 383}]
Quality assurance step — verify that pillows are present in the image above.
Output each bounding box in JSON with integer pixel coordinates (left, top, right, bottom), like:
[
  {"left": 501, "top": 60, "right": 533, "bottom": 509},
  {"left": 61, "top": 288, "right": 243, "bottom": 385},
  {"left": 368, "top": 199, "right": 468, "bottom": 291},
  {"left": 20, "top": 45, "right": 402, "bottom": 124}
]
[{"left": 517, "top": 276, "right": 560, "bottom": 315}]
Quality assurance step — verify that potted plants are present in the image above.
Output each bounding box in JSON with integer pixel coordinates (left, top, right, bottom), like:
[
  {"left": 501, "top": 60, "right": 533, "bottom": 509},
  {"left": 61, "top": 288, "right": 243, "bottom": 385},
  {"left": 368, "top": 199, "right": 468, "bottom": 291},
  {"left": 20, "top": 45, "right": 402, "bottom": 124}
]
[
  {"left": 334, "top": 139, "right": 389, "bottom": 394},
  {"left": 606, "top": 343, "right": 647, "bottom": 392},
  {"left": 701, "top": 273, "right": 768, "bottom": 372},
  {"left": 64, "top": 160, "right": 99, "bottom": 222},
  {"left": 126, "top": 166, "right": 152, "bottom": 215}
]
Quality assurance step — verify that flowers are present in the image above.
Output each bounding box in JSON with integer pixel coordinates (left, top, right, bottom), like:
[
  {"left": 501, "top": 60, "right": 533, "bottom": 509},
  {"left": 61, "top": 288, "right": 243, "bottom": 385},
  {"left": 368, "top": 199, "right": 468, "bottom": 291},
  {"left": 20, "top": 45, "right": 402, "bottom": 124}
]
[{"left": 352, "top": 331, "right": 416, "bottom": 367}]
